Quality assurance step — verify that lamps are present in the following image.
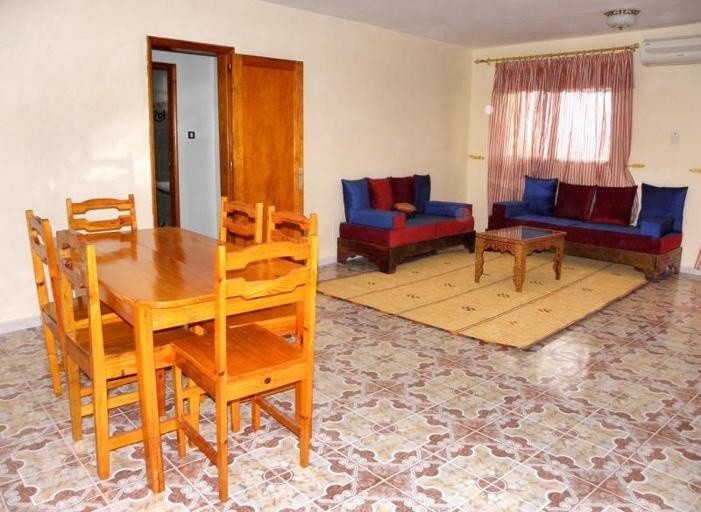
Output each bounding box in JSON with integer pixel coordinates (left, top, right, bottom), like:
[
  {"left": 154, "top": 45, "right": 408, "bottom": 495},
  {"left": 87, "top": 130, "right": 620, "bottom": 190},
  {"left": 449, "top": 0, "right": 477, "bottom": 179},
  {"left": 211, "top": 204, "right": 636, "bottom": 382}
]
[{"left": 604, "top": 9, "right": 639, "bottom": 31}]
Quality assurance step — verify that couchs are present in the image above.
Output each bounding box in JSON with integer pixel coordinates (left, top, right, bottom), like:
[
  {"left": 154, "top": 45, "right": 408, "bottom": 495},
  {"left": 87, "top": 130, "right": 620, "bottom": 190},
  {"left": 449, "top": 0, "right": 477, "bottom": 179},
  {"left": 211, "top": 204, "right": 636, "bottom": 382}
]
[
  {"left": 488, "top": 200, "right": 682, "bottom": 280},
  {"left": 336, "top": 200, "right": 476, "bottom": 273}
]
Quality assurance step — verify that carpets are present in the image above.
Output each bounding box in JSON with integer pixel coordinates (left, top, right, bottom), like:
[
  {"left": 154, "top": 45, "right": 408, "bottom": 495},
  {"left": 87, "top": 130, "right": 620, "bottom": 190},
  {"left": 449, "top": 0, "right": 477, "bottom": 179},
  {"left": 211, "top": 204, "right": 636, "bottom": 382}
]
[{"left": 316, "top": 245, "right": 649, "bottom": 348}]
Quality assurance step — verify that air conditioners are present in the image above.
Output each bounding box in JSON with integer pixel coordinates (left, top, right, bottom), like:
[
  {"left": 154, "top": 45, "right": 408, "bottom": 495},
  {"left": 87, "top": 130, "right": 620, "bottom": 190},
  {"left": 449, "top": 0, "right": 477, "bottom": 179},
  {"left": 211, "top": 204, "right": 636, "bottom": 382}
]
[{"left": 638, "top": 35, "right": 701, "bottom": 66}]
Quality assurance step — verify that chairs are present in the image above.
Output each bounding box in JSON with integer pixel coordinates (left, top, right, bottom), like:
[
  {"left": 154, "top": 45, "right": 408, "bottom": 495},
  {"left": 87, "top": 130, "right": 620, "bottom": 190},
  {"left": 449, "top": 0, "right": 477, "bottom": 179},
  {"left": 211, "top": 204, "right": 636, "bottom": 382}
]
[
  {"left": 170, "top": 237, "right": 318, "bottom": 501},
  {"left": 67, "top": 194, "right": 137, "bottom": 232},
  {"left": 54, "top": 229, "right": 186, "bottom": 477},
  {"left": 219, "top": 197, "right": 263, "bottom": 250},
  {"left": 191, "top": 206, "right": 318, "bottom": 431},
  {"left": 27, "top": 210, "right": 117, "bottom": 395}
]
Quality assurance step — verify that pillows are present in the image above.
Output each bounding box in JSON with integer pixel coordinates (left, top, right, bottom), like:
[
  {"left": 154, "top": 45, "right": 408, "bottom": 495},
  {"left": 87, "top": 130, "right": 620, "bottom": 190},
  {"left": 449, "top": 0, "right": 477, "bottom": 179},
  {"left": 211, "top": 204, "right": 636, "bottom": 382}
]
[
  {"left": 590, "top": 187, "right": 637, "bottom": 226},
  {"left": 637, "top": 184, "right": 689, "bottom": 232},
  {"left": 554, "top": 182, "right": 597, "bottom": 220},
  {"left": 524, "top": 175, "right": 557, "bottom": 217},
  {"left": 342, "top": 178, "right": 368, "bottom": 224},
  {"left": 391, "top": 176, "right": 413, "bottom": 206},
  {"left": 413, "top": 174, "right": 430, "bottom": 213},
  {"left": 368, "top": 178, "right": 393, "bottom": 210}
]
